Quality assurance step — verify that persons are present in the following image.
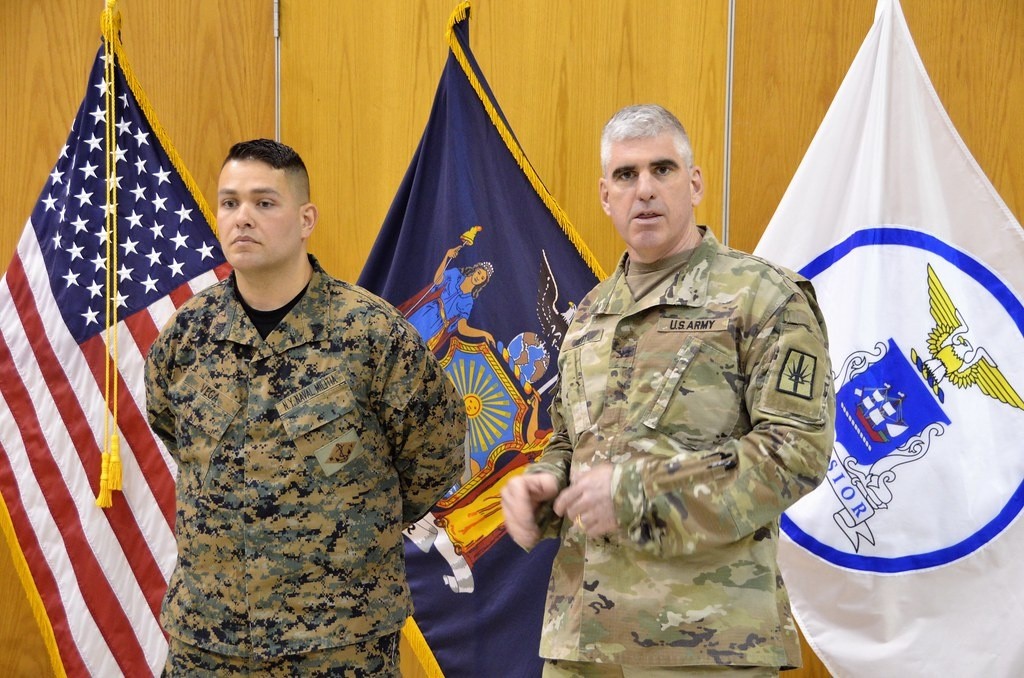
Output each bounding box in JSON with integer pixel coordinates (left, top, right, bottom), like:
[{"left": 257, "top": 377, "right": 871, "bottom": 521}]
[
  {"left": 144, "top": 138, "right": 465, "bottom": 678},
  {"left": 499, "top": 102, "right": 834, "bottom": 678}
]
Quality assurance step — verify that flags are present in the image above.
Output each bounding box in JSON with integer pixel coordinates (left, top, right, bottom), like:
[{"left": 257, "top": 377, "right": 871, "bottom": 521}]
[
  {"left": 356, "top": 3, "right": 610, "bottom": 678},
  {"left": 0, "top": 2, "right": 232, "bottom": 678},
  {"left": 753, "top": 0, "right": 1024, "bottom": 678}
]
